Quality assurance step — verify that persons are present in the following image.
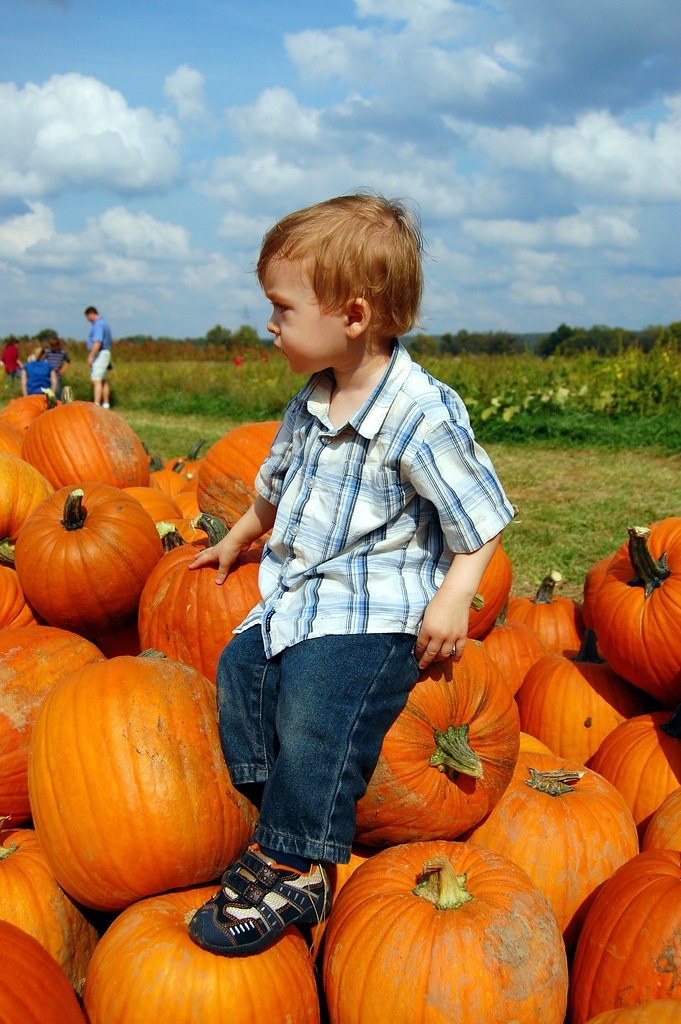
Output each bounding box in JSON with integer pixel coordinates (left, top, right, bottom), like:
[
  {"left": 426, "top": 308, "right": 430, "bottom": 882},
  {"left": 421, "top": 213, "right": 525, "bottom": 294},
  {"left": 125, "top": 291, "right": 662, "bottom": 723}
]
[
  {"left": 45, "top": 338, "right": 72, "bottom": 398},
  {"left": 85, "top": 307, "right": 111, "bottom": 409},
  {"left": 187, "top": 194, "right": 514, "bottom": 958},
  {"left": 22, "top": 348, "right": 57, "bottom": 396},
  {"left": 0, "top": 339, "right": 23, "bottom": 384}
]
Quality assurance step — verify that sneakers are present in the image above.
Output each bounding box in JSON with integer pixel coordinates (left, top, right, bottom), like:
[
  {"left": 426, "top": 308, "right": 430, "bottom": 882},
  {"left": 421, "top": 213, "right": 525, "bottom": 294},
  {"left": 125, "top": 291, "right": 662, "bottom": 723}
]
[{"left": 185, "top": 840, "right": 336, "bottom": 958}]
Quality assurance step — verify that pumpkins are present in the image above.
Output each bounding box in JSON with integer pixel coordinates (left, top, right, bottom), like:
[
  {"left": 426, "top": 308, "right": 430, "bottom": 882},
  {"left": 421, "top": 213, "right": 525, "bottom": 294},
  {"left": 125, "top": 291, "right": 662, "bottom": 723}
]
[{"left": 0, "top": 385, "right": 681, "bottom": 1024}]
